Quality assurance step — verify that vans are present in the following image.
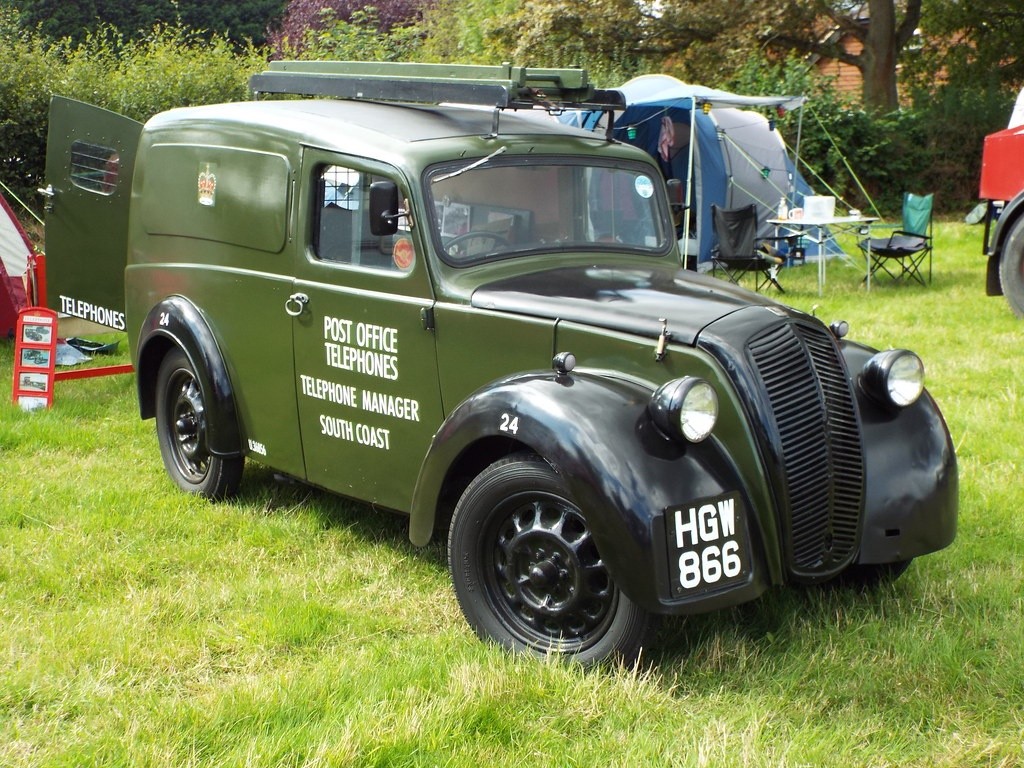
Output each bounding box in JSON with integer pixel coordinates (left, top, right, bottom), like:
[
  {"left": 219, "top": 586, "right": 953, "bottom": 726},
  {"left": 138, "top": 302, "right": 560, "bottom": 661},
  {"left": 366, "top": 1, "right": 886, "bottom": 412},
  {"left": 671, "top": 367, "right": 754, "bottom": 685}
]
[{"left": 46, "top": 61, "right": 960, "bottom": 684}]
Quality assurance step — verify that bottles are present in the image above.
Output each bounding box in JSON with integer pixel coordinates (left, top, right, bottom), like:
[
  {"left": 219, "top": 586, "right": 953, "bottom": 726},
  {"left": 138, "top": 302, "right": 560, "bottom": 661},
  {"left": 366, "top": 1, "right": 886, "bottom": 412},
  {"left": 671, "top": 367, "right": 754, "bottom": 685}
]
[{"left": 778, "top": 197, "right": 787, "bottom": 221}]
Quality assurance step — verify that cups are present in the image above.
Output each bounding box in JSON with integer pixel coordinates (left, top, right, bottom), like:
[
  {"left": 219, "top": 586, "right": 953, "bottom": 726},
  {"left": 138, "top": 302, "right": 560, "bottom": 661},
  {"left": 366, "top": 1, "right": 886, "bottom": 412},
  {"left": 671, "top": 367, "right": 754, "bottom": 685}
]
[{"left": 789, "top": 208, "right": 804, "bottom": 221}]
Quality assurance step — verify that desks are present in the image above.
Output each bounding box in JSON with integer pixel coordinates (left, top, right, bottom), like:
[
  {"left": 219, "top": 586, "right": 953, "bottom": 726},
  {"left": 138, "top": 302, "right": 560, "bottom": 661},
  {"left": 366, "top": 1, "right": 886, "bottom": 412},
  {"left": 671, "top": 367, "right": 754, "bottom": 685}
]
[{"left": 766, "top": 217, "right": 881, "bottom": 296}]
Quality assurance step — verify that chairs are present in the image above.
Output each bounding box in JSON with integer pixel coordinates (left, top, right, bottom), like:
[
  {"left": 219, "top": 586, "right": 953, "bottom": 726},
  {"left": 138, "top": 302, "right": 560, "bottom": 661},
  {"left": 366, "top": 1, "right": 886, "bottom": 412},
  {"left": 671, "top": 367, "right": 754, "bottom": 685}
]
[
  {"left": 858, "top": 191, "right": 936, "bottom": 288},
  {"left": 710, "top": 204, "right": 793, "bottom": 293}
]
